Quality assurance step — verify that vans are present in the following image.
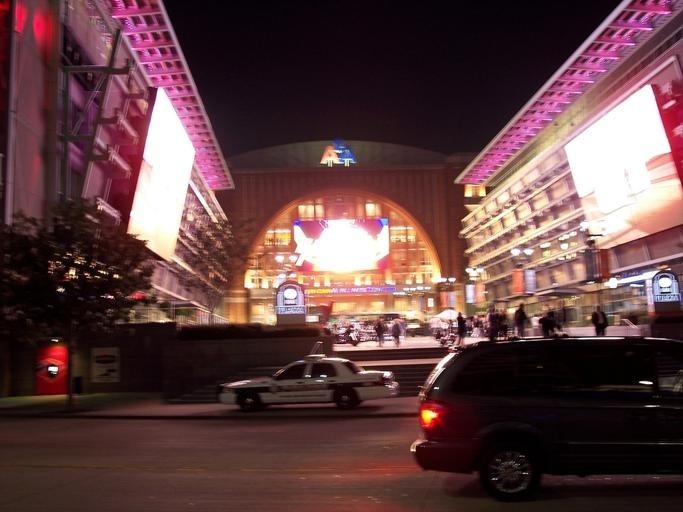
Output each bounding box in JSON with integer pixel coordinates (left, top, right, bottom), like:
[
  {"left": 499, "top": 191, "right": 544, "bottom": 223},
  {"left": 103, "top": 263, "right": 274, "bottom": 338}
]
[{"left": 409, "top": 338, "right": 683, "bottom": 503}]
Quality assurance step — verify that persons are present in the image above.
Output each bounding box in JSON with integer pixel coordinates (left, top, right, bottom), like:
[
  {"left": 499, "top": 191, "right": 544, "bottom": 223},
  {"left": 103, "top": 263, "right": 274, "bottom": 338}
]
[
  {"left": 592, "top": 305, "right": 608, "bottom": 337},
  {"left": 429, "top": 304, "right": 562, "bottom": 347},
  {"left": 319, "top": 316, "right": 405, "bottom": 347}
]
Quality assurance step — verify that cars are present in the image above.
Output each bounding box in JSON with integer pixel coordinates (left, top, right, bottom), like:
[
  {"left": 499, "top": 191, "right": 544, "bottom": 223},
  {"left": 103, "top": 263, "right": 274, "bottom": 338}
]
[{"left": 219, "top": 353, "right": 399, "bottom": 413}]
